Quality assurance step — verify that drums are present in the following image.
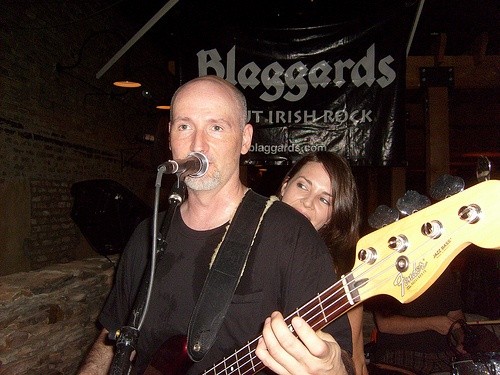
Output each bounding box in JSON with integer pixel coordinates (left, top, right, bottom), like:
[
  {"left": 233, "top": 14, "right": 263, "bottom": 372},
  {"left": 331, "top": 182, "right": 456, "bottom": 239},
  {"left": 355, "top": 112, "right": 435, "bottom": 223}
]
[{"left": 449, "top": 352, "right": 500, "bottom": 375}]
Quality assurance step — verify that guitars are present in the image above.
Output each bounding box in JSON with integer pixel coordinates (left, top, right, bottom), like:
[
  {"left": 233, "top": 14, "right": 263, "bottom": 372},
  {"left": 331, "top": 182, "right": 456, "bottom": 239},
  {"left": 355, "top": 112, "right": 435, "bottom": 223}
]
[{"left": 200, "top": 180, "right": 500, "bottom": 375}]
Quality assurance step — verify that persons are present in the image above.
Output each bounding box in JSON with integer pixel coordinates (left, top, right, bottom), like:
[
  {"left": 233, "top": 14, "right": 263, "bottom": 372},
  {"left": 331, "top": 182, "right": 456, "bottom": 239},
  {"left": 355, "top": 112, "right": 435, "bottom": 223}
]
[
  {"left": 74, "top": 75, "right": 356, "bottom": 375},
  {"left": 370, "top": 275, "right": 472, "bottom": 375},
  {"left": 275, "top": 151, "right": 369, "bottom": 375}
]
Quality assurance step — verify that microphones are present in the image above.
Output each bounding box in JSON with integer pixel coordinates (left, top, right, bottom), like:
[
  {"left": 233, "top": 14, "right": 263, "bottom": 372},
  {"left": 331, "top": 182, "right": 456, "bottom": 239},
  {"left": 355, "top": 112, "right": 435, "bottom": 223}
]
[
  {"left": 157, "top": 152, "right": 208, "bottom": 178},
  {"left": 458, "top": 319, "right": 479, "bottom": 346}
]
